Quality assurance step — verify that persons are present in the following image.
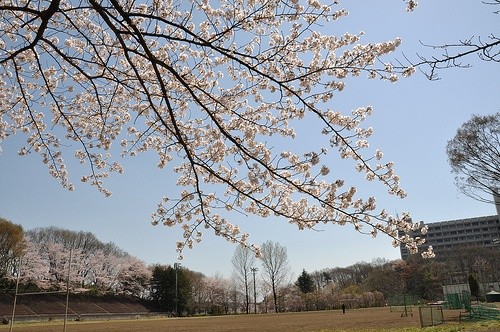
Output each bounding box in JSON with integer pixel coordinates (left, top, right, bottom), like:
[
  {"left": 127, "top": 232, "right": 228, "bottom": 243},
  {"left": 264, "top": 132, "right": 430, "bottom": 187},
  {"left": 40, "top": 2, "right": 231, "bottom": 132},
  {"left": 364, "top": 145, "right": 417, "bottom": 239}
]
[{"left": 342, "top": 303, "right": 346, "bottom": 314}]
